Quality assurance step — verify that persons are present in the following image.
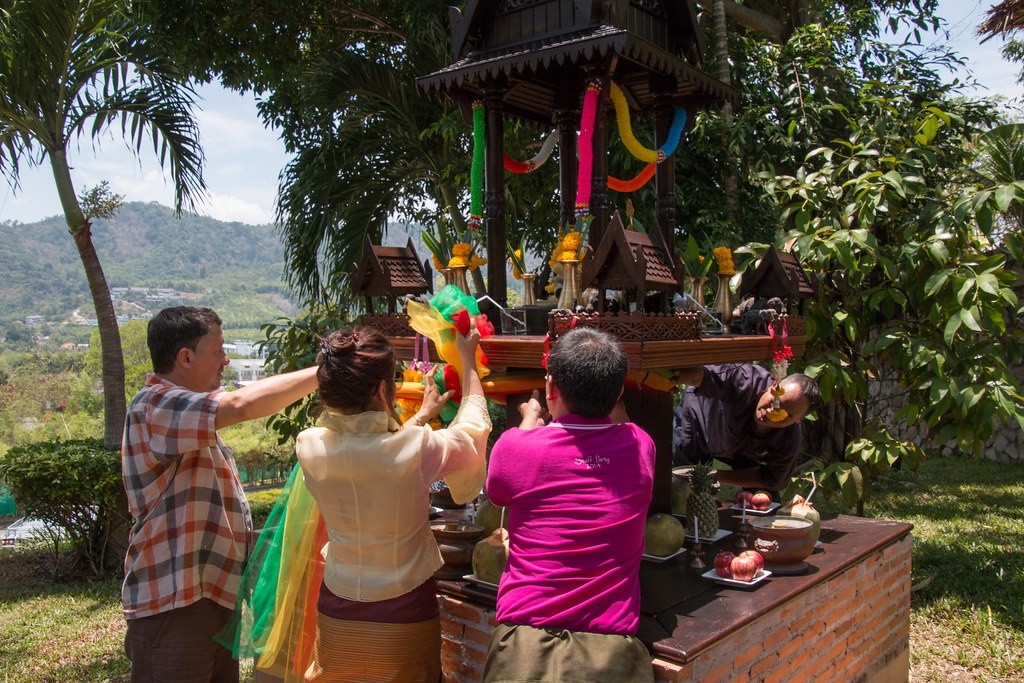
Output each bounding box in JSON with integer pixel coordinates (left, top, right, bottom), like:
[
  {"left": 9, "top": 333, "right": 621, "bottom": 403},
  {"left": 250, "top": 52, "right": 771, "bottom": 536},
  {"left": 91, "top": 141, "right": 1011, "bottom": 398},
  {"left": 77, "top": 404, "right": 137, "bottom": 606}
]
[
  {"left": 119, "top": 306, "right": 322, "bottom": 683},
  {"left": 484, "top": 329, "right": 660, "bottom": 683},
  {"left": 674, "top": 361, "right": 822, "bottom": 509},
  {"left": 296, "top": 319, "right": 493, "bottom": 682}
]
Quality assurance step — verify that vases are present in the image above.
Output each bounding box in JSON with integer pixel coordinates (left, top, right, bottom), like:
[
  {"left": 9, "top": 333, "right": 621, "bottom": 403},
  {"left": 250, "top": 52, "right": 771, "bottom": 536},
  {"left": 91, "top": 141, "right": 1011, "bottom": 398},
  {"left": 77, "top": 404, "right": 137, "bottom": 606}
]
[
  {"left": 521, "top": 273, "right": 540, "bottom": 307},
  {"left": 712, "top": 273, "right": 734, "bottom": 332},
  {"left": 556, "top": 260, "right": 580, "bottom": 315},
  {"left": 449, "top": 265, "right": 471, "bottom": 297},
  {"left": 436, "top": 268, "right": 454, "bottom": 288},
  {"left": 688, "top": 276, "right": 709, "bottom": 338}
]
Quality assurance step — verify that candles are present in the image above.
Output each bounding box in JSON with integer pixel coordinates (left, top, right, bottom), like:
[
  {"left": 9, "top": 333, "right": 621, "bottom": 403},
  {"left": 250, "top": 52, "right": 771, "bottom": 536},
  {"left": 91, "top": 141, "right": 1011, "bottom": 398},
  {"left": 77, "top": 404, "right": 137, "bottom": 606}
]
[
  {"left": 742, "top": 498, "right": 746, "bottom": 526},
  {"left": 693, "top": 515, "right": 699, "bottom": 543}
]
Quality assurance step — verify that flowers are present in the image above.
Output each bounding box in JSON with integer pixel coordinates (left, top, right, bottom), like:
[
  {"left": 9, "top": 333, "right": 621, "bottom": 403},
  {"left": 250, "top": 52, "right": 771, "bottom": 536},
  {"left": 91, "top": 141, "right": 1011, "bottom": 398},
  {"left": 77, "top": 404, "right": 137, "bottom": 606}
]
[
  {"left": 761, "top": 359, "right": 792, "bottom": 423},
  {"left": 415, "top": 213, "right": 758, "bottom": 293},
  {"left": 400, "top": 368, "right": 441, "bottom": 431}
]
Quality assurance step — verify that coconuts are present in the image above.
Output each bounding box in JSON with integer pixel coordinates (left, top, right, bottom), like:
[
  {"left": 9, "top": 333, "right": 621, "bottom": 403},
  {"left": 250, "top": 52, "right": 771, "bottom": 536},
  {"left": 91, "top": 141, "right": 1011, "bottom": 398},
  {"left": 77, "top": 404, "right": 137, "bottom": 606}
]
[
  {"left": 646, "top": 515, "right": 685, "bottom": 556},
  {"left": 777, "top": 495, "right": 820, "bottom": 544},
  {"left": 473, "top": 529, "right": 509, "bottom": 586}
]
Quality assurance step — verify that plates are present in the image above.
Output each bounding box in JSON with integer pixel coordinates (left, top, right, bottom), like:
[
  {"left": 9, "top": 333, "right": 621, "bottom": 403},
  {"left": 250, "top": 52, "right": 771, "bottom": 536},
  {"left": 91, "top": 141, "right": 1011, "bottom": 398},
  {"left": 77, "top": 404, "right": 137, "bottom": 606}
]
[
  {"left": 730, "top": 502, "right": 781, "bottom": 515},
  {"left": 702, "top": 568, "right": 772, "bottom": 588},
  {"left": 461, "top": 574, "right": 499, "bottom": 591},
  {"left": 642, "top": 547, "right": 687, "bottom": 563},
  {"left": 685, "top": 528, "right": 733, "bottom": 544}
]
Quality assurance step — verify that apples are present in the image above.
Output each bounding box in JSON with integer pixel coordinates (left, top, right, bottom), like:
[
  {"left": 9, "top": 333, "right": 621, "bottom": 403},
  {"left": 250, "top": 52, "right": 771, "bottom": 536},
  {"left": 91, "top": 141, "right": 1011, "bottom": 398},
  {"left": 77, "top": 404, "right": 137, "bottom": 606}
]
[
  {"left": 738, "top": 491, "right": 771, "bottom": 510},
  {"left": 715, "top": 550, "right": 764, "bottom": 582}
]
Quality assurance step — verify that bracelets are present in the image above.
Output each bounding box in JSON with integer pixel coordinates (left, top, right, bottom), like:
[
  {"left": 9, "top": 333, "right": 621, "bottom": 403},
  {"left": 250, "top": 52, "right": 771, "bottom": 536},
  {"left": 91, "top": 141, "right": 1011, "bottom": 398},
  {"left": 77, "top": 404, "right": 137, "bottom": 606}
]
[{"left": 612, "top": 415, "right": 630, "bottom": 422}]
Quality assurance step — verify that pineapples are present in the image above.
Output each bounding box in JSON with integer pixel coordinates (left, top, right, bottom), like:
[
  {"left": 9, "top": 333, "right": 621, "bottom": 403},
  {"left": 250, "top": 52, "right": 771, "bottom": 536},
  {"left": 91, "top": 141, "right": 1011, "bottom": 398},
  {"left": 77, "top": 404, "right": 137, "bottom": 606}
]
[{"left": 685, "top": 462, "right": 720, "bottom": 537}]
